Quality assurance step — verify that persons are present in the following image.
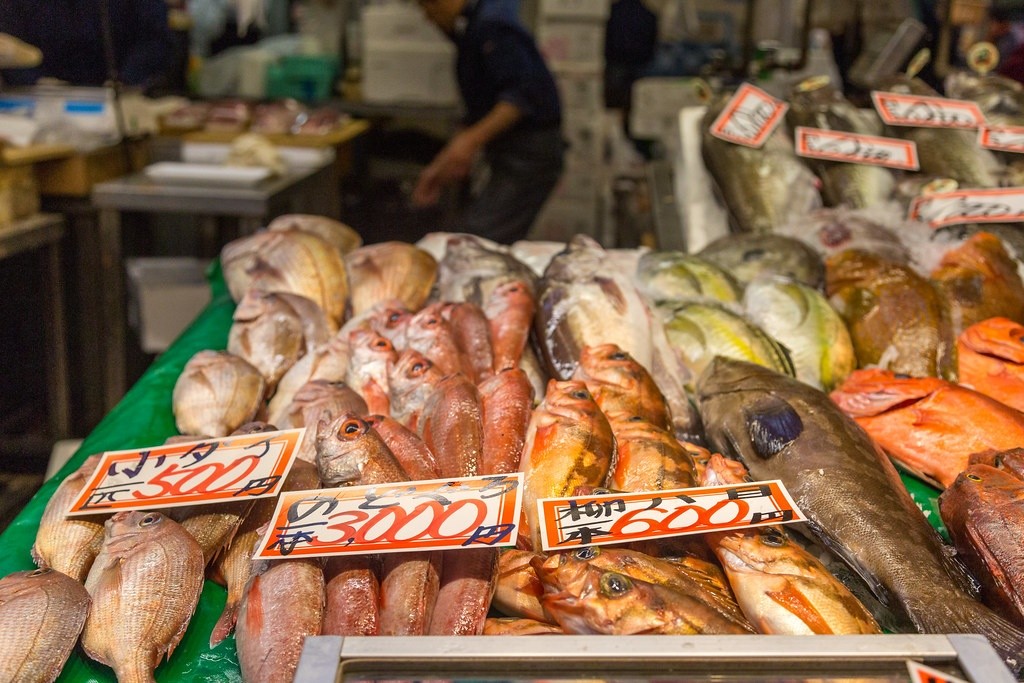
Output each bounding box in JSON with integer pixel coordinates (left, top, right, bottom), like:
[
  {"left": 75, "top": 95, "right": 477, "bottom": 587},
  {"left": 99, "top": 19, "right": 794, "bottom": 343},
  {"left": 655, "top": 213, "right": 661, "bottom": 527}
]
[
  {"left": 603, "top": 1, "right": 657, "bottom": 163},
  {"left": 833, "top": 1, "right": 1024, "bottom": 109},
  {"left": 2, "top": 0, "right": 189, "bottom": 475},
  {"left": 411, "top": 0, "right": 569, "bottom": 246}
]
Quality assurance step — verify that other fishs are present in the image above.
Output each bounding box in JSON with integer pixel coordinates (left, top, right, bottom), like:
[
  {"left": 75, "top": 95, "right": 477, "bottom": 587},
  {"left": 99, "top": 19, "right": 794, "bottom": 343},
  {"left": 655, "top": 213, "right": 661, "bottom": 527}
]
[
  {"left": 0, "top": 212, "right": 1024, "bottom": 683},
  {"left": 693, "top": 65, "right": 1024, "bottom": 234}
]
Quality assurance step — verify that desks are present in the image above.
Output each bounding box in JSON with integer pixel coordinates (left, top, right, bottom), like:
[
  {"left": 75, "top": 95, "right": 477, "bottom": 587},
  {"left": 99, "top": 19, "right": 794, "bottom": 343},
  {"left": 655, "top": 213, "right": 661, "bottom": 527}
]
[{"left": 0, "top": 103, "right": 369, "bottom": 475}]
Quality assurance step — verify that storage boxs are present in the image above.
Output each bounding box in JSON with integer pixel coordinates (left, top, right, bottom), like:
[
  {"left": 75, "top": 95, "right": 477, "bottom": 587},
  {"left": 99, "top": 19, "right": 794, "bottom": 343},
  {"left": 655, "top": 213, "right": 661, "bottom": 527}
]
[
  {"left": 677, "top": 97, "right": 885, "bottom": 265},
  {"left": 262, "top": 54, "right": 345, "bottom": 108},
  {"left": 629, "top": 74, "right": 729, "bottom": 143},
  {"left": 360, "top": 3, "right": 462, "bottom": 110},
  {"left": 520, "top": 0, "right": 616, "bottom": 250}
]
